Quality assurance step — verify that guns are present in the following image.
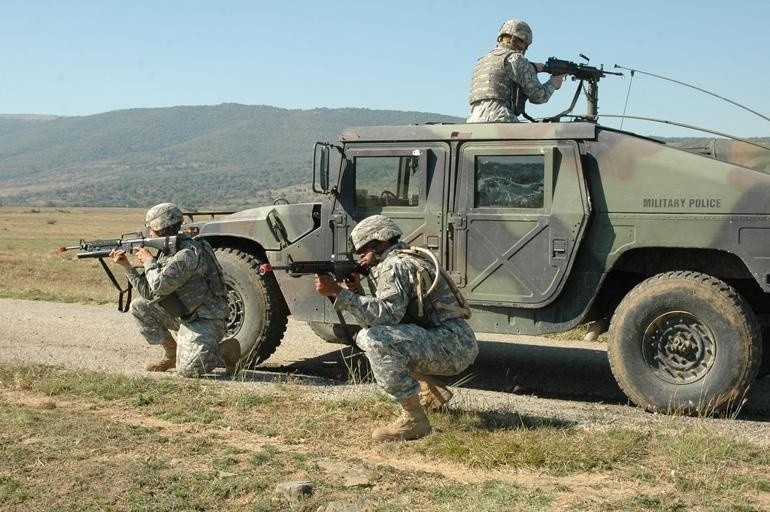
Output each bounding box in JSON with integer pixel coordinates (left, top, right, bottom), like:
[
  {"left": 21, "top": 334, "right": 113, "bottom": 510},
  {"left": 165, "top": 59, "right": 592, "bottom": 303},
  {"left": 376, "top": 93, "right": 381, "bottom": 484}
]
[
  {"left": 259, "top": 253, "right": 372, "bottom": 293},
  {"left": 59, "top": 231, "right": 180, "bottom": 259},
  {"left": 529, "top": 53, "right": 623, "bottom": 89}
]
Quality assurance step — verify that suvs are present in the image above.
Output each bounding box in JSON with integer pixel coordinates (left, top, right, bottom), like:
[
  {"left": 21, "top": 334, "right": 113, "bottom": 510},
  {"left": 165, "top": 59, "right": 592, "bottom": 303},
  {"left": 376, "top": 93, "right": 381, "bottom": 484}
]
[{"left": 178, "top": 121, "right": 770, "bottom": 415}]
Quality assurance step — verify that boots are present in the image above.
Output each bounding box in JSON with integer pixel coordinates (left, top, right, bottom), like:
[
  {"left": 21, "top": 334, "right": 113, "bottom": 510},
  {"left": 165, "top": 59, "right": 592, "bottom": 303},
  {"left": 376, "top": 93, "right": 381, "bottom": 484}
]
[
  {"left": 219, "top": 339, "right": 241, "bottom": 378},
  {"left": 372, "top": 373, "right": 453, "bottom": 439},
  {"left": 144, "top": 337, "right": 176, "bottom": 371}
]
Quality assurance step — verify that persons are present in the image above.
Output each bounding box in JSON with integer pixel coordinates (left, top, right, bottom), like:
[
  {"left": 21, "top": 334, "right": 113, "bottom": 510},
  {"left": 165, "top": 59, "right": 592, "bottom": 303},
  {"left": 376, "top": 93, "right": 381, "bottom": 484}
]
[
  {"left": 465, "top": 19, "right": 563, "bottom": 123},
  {"left": 108, "top": 202, "right": 242, "bottom": 381},
  {"left": 314, "top": 214, "right": 480, "bottom": 442}
]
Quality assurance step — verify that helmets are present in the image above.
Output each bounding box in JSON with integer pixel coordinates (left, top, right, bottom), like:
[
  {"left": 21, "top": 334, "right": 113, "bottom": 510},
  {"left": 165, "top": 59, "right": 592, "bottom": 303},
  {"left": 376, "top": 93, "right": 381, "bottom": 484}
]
[
  {"left": 145, "top": 203, "right": 184, "bottom": 231},
  {"left": 497, "top": 20, "right": 532, "bottom": 46},
  {"left": 348, "top": 215, "right": 402, "bottom": 252}
]
